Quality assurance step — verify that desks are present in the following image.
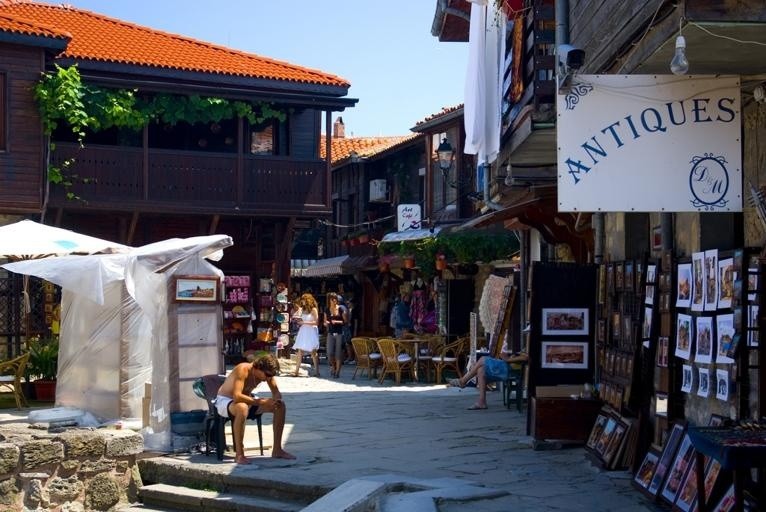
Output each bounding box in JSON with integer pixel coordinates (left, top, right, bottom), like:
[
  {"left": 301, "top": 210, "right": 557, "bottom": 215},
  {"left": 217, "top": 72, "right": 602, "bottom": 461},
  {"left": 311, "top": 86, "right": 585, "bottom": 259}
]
[{"left": 687, "top": 423, "right": 766, "bottom": 512}]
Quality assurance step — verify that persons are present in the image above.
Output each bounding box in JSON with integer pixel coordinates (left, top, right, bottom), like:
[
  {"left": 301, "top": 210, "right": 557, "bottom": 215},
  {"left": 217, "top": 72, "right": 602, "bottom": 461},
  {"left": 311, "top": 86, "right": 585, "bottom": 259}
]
[
  {"left": 386, "top": 283, "right": 440, "bottom": 385},
  {"left": 446, "top": 345, "right": 527, "bottom": 409},
  {"left": 288, "top": 281, "right": 359, "bottom": 377},
  {"left": 215, "top": 354, "right": 297, "bottom": 464}
]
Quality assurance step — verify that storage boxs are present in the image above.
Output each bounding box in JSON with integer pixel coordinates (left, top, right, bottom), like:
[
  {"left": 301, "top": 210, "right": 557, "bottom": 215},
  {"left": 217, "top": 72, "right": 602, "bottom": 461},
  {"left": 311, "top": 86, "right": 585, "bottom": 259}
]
[{"left": 530, "top": 396, "right": 606, "bottom": 442}]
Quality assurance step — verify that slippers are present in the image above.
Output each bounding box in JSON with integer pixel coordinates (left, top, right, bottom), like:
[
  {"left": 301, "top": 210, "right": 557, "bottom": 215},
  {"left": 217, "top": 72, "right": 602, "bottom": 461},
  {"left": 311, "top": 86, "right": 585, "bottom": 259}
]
[
  {"left": 446, "top": 376, "right": 466, "bottom": 389},
  {"left": 467, "top": 403, "right": 487, "bottom": 410}
]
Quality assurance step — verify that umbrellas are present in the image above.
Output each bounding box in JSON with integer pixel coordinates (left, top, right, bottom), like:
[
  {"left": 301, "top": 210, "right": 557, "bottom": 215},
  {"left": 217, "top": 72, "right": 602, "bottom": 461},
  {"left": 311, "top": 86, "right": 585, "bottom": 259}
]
[{"left": 0, "top": 218, "right": 126, "bottom": 400}]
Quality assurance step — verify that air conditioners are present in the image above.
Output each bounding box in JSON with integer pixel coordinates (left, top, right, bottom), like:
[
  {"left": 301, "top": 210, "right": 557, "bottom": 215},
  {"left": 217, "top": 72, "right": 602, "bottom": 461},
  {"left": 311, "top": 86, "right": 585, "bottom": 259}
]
[{"left": 369, "top": 179, "right": 387, "bottom": 203}]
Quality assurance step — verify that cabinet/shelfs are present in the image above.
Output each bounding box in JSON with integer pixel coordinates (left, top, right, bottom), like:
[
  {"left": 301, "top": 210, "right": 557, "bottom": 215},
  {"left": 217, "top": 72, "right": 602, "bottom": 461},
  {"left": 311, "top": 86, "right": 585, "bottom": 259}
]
[{"left": 222, "top": 269, "right": 254, "bottom": 360}]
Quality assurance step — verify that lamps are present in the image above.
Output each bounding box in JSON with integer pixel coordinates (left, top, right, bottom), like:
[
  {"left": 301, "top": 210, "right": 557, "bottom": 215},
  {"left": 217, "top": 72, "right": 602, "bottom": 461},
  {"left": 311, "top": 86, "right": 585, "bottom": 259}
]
[
  {"left": 670, "top": 35, "right": 689, "bottom": 76},
  {"left": 504, "top": 164, "right": 515, "bottom": 187},
  {"left": 435, "top": 136, "right": 474, "bottom": 189}
]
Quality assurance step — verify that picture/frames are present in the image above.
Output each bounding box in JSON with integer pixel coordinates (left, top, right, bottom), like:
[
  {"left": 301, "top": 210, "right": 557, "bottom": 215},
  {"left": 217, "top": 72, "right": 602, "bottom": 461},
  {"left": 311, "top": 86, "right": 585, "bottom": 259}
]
[
  {"left": 586, "top": 258, "right": 647, "bottom": 469},
  {"left": 657, "top": 336, "right": 670, "bottom": 370},
  {"left": 171, "top": 275, "right": 221, "bottom": 306},
  {"left": 652, "top": 224, "right": 662, "bottom": 251},
  {"left": 631, "top": 414, "right": 736, "bottom": 512},
  {"left": 653, "top": 390, "right": 669, "bottom": 420}
]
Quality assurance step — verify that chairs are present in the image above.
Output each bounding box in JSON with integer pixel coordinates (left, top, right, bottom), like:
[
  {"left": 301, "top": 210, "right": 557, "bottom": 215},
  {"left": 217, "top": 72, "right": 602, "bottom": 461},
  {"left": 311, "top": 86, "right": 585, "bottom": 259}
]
[
  {"left": 515, "top": 360, "right": 530, "bottom": 413},
  {"left": 502, "top": 352, "right": 524, "bottom": 409},
  {"left": 0, "top": 351, "right": 31, "bottom": 411},
  {"left": 192, "top": 374, "right": 264, "bottom": 460},
  {"left": 350, "top": 332, "right": 487, "bottom": 387}
]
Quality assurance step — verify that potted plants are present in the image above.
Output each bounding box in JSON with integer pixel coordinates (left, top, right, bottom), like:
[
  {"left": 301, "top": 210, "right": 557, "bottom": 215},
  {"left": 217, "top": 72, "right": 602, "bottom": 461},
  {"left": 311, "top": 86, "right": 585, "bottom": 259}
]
[
  {"left": 428, "top": 236, "right": 449, "bottom": 271},
  {"left": 22, "top": 339, "right": 61, "bottom": 402},
  {"left": 377, "top": 241, "right": 389, "bottom": 272},
  {"left": 17, "top": 348, "right": 36, "bottom": 400},
  {"left": 339, "top": 228, "right": 371, "bottom": 248},
  {"left": 400, "top": 239, "right": 416, "bottom": 269}
]
